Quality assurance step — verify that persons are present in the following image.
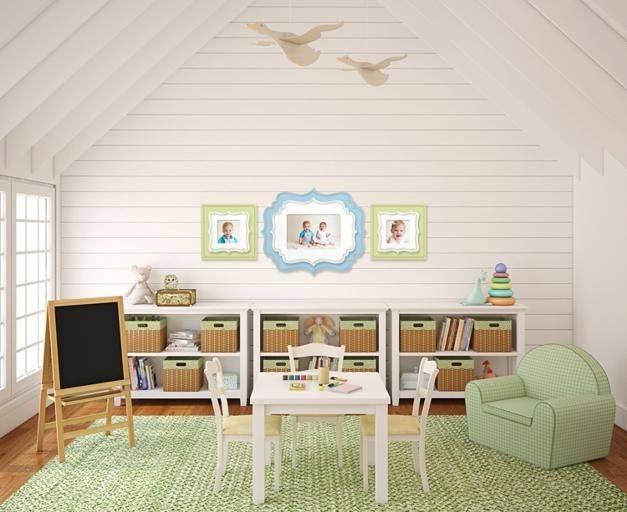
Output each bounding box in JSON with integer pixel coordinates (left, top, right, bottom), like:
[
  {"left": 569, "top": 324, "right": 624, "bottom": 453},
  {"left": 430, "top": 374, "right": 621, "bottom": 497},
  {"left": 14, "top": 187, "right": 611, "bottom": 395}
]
[
  {"left": 313, "top": 222, "right": 334, "bottom": 246},
  {"left": 218, "top": 222, "right": 240, "bottom": 243},
  {"left": 387, "top": 220, "right": 408, "bottom": 244},
  {"left": 299, "top": 221, "right": 317, "bottom": 246}
]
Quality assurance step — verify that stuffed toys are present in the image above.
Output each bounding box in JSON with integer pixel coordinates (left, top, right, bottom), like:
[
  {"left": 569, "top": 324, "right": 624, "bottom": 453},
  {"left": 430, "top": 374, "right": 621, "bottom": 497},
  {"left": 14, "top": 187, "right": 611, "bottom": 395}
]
[{"left": 304, "top": 315, "right": 336, "bottom": 344}]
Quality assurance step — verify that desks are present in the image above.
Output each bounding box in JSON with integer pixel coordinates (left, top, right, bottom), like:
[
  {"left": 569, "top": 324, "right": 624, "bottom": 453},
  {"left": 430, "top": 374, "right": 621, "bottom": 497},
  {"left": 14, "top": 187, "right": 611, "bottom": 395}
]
[{"left": 244, "top": 368, "right": 394, "bottom": 505}]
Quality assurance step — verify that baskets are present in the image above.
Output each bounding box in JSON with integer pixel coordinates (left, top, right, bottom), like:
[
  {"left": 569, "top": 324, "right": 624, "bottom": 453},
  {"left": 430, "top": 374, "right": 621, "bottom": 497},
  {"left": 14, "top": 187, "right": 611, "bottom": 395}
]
[
  {"left": 469, "top": 316, "right": 513, "bottom": 352},
  {"left": 263, "top": 317, "right": 300, "bottom": 352},
  {"left": 200, "top": 316, "right": 240, "bottom": 353},
  {"left": 163, "top": 356, "right": 204, "bottom": 392},
  {"left": 339, "top": 316, "right": 377, "bottom": 353},
  {"left": 343, "top": 357, "right": 376, "bottom": 372},
  {"left": 400, "top": 317, "right": 437, "bottom": 351},
  {"left": 433, "top": 357, "right": 474, "bottom": 392},
  {"left": 263, "top": 357, "right": 299, "bottom": 372},
  {"left": 125, "top": 316, "right": 167, "bottom": 352}
]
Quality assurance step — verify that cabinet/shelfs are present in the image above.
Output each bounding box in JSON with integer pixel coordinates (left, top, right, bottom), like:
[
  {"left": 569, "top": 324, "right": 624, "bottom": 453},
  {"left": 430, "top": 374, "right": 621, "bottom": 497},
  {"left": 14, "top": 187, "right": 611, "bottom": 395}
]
[
  {"left": 247, "top": 299, "right": 392, "bottom": 407},
  {"left": 106, "top": 299, "right": 252, "bottom": 410},
  {"left": 387, "top": 301, "right": 528, "bottom": 410}
]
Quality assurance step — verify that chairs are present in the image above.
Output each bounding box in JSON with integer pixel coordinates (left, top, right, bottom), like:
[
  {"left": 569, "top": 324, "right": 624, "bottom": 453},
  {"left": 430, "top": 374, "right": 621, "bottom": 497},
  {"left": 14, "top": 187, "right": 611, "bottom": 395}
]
[
  {"left": 285, "top": 341, "right": 346, "bottom": 469},
  {"left": 357, "top": 356, "right": 441, "bottom": 498},
  {"left": 202, "top": 356, "right": 284, "bottom": 498},
  {"left": 461, "top": 340, "right": 616, "bottom": 472}
]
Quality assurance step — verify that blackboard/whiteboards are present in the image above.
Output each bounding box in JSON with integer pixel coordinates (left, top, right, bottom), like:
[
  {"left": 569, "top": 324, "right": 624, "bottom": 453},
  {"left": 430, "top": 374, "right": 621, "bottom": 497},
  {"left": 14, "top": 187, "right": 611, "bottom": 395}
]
[{"left": 48, "top": 296, "right": 131, "bottom": 397}]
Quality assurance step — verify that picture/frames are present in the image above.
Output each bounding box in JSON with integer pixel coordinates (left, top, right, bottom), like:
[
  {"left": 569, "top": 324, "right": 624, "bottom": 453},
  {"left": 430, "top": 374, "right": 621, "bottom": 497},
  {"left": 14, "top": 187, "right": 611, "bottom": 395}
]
[
  {"left": 369, "top": 203, "right": 429, "bottom": 263},
  {"left": 198, "top": 203, "right": 258, "bottom": 261},
  {"left": 259, "top": 187, "right": 368, "bottom": 277}
]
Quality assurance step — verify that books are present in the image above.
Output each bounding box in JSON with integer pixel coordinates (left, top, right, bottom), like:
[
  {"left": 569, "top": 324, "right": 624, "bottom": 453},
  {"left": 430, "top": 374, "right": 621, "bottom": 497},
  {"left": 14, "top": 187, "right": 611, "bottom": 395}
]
[
  {"left": 165, "top": 329, "right": 201, "bottom": 352},
  {"left": 308, "top": 357, "right": 338, "bottom": 371},
  {"left": 437, "top": 316, "right": 475, "bottom": 352},
  {"left": 128, "top": 356, "right": 157, "bottom": 390},
  {"left": 327, "top": 382, "right": 362, "bottom": 394}
]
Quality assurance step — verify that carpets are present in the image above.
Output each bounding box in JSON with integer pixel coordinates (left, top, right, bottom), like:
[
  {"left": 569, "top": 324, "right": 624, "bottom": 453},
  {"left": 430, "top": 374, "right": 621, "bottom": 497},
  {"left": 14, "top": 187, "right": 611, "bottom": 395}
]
[{"left": 1, "top": 409, "right": 626, "bottom": 512}]
